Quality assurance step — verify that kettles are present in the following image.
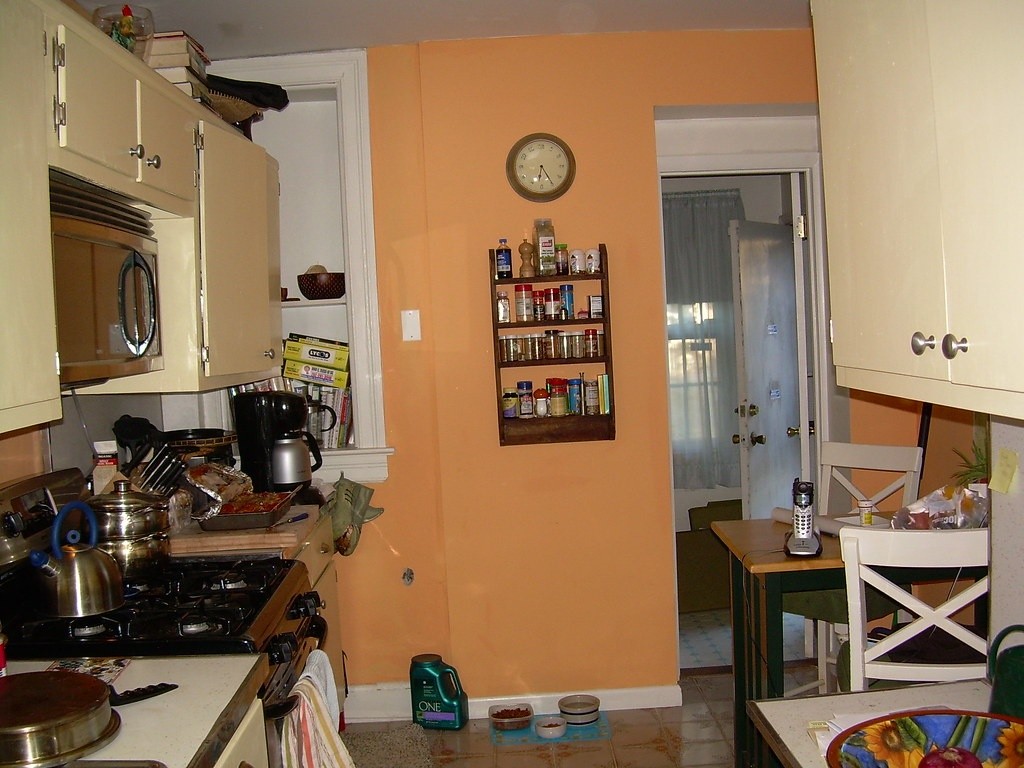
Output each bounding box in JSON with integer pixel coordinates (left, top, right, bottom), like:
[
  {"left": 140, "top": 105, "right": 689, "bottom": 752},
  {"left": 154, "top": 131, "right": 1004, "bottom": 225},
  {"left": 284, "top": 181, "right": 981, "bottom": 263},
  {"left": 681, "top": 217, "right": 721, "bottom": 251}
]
[{"left": 30, "top": 498, "right": 126, "bottom": 618}]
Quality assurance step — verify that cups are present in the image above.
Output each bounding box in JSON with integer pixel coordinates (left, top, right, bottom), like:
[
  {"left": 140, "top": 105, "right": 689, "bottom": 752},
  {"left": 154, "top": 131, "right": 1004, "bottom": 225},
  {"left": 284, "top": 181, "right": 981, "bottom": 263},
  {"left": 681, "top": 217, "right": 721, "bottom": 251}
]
[{"left": 281, "top": 287, "right": 287, "bottom": 300}]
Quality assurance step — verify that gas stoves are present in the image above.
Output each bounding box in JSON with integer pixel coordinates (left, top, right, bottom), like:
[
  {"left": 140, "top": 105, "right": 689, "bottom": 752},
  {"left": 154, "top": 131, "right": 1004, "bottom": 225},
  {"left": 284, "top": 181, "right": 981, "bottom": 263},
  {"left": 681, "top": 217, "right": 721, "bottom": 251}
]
[{"left": 0, "top": 467, "right": 320, "bottom": 698}]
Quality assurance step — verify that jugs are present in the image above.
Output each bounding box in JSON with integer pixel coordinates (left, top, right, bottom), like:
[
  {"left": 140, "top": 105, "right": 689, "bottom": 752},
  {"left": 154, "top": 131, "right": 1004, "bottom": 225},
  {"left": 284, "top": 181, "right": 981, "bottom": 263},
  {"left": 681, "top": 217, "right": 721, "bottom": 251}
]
[
  {"left": 300, "top": 395, "right": 338, "bottom": 450},
  {"left": 272, "top": 431, "right": 323, "bottom": 492}
]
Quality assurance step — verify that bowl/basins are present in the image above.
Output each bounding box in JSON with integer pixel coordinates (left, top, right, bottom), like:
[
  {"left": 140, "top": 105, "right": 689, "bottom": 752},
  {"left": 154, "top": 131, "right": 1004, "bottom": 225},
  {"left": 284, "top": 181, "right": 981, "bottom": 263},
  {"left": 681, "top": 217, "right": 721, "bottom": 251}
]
[
  {"left": 558, "top": 694, "right": 601, "bottom": 726},
  {"left": 489, "top": 703, "right": 534, "bottom": 730},
  {"left": 535, "top": 716, "right": 569, "bottom": 738},
  {"left": 298, "top": 272, "right": 346, "bottom": 300}
]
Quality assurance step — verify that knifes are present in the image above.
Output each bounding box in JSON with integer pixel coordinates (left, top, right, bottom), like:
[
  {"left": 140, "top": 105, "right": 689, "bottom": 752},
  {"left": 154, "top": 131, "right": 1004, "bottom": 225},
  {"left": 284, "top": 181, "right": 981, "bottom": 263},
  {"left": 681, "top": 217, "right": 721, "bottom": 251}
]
[
  {"left": 120, "top": 440, "right": 189, "bottom": 498},
  {"left": 266, "top": 513, "right": 309, "bottom": 533}
]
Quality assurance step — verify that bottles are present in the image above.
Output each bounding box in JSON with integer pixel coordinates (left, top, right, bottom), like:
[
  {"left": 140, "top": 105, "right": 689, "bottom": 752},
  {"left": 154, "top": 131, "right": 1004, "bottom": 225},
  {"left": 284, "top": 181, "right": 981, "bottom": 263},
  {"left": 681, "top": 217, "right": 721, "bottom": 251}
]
[
  {"left": 496, "top": 219, "right": 601, "bottom": 278},
  {"left": 496, "top": 285, "right": 576, "bottom": 322},
  {"left": 499, "top": 329, "right": 606, "bottom": 362},
  {"left": 502, "top": 372, "right": 600, "bottom": 418},
  {"left": 409, "top": 653, "right": 469, "bottom": 730},
  {"left": 858, "top": 499, "right": 874, "bottom": 526}
]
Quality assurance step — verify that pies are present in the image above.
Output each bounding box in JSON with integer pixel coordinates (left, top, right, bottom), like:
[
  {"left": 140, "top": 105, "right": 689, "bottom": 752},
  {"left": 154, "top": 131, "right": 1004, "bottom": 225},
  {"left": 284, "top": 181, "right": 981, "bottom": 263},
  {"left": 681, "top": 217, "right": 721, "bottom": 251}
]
[{"left": 221, "top": 493, "right": 292, "bottom": 512}]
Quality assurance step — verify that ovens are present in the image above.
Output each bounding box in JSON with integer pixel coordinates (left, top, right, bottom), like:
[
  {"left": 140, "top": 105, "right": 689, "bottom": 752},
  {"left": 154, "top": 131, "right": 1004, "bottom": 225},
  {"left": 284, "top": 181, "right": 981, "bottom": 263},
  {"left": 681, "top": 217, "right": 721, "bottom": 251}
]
[{"left": 262, "top": 614, "right": 328, "bottom": 768}]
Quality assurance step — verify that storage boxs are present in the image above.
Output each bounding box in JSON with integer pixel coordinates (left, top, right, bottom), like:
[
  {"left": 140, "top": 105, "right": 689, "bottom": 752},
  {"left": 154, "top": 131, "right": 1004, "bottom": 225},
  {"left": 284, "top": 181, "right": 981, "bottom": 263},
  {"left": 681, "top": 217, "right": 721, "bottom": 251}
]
[{"left": 283, "top": 340, "right": 349, "bottom": 388}]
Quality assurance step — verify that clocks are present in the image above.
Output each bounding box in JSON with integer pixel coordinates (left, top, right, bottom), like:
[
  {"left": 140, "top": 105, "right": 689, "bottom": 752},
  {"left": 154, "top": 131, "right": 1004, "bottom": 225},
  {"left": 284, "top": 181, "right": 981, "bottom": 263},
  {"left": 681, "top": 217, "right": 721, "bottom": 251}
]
[{"left": 505, "top": 132, "right": 577, "bottom": 203}]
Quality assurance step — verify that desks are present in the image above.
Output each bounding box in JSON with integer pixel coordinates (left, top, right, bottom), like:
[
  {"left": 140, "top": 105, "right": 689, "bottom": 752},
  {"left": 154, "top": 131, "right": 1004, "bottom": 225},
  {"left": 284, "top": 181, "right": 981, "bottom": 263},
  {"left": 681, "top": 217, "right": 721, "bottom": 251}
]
[
  {"left": 746, "top": 677, "right": 1024, "bottom": 768},
  {"left": 708, "top": 510, "right": 991, "bottom": 768}
]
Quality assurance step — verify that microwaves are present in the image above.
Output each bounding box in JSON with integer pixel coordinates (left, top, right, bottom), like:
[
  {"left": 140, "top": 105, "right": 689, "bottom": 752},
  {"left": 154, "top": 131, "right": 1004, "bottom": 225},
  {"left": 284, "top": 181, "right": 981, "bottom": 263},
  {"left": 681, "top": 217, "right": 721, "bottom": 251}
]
[{"left": 49, "top": 181, "right": 166, "bottom": 391}]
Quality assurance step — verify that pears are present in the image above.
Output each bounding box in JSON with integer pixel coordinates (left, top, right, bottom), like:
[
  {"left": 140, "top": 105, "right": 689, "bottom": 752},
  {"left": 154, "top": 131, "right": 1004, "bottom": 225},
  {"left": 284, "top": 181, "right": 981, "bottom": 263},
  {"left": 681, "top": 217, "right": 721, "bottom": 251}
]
[{"left": 304, "top": 265, "right": 328, "bottom": 274}]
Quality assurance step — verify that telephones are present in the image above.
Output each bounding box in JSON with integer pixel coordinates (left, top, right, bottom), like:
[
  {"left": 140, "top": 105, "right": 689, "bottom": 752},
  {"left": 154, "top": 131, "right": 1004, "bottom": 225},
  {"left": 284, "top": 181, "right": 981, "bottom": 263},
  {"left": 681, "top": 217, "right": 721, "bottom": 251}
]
[{"left": 784, "top": 478, "right": 823, "bottom": 558}]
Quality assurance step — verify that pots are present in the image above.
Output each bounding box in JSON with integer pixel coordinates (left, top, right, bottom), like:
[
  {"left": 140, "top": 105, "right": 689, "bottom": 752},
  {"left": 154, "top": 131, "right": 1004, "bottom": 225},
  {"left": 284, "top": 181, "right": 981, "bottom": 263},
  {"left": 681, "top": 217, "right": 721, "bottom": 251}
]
[
  {"left": 80, "top": 480, "right": 171, "bottom": 574},
  {"left": 0, "top": 670, "right": 180, "bottom": 767}
]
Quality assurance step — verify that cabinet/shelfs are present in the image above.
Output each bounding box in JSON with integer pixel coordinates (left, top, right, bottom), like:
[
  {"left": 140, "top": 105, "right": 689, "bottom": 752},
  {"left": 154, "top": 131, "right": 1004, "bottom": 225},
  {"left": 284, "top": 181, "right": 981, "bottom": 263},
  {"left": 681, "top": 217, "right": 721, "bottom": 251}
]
[
  {"left": 809, "top": 0, "right": 1024, "bottom": 420},
  {"left": 0, "top": 0, "right": 63, "bottom": 435},
  {"left": 60, "top": 100, "right": 283, "bottom": 396},
  {"left": 53, "top": 3, "right": 195, "bottom": 219},
  {"left": 206, "top": 48, "right": 395, "bottom": 486},
  {"left": 279, "top": 505, "right": 348, "bottom": 733},
  {"left": 488, "top": 243, "right": 616, "bottom": 446}
]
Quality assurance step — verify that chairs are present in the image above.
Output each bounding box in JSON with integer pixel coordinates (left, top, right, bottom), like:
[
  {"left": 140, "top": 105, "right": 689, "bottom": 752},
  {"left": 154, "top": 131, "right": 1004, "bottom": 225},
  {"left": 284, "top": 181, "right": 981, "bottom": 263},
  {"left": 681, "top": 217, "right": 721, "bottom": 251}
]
[
  {"left": 840, "top": 525, "right": 994, "bottom": 692},
  {"left": 779, "top": 440, "right": 924, "bottom": 698}
]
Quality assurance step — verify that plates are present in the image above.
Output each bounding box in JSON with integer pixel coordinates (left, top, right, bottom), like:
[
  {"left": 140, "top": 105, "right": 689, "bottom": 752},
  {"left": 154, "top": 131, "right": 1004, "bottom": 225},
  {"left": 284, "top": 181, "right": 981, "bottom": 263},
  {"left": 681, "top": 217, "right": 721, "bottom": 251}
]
[
  {"left": 833, "top": 515, "right": 891, "bottom": 529},
  {"left": 827, "top": 710, "right": 1024, "bottom": 768}
]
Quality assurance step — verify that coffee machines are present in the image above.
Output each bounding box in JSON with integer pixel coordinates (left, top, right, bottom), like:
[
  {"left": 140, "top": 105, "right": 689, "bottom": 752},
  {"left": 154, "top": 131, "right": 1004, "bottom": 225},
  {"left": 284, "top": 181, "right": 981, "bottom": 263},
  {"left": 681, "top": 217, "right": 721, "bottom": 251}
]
[{"left": 234, "top": 390, "right": 327, "bottom": 507}]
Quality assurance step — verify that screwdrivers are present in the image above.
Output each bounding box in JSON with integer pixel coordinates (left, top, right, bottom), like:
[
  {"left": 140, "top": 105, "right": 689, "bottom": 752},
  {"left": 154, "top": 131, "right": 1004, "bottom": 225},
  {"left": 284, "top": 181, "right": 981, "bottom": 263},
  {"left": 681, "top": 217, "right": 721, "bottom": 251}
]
[{"left": 270, "top": 512, "right": 309, "bottom": 528}]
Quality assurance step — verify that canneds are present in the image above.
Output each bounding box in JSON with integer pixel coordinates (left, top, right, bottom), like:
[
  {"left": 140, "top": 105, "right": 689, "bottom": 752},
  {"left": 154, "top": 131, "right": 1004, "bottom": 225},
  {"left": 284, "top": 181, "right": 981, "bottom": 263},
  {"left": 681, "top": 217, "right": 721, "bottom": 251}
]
[
  {"left": 554, "top": 244, "right": 569, "bottom": 276},
  {"left": 500, "top": 328, "right": 605, "bottom": 363},
  {"left": 514, "top": 284, "right": 575, "bottom": 321},
  {"left": 502, "top": 378, "right": 599, "bottom": 419}
]
